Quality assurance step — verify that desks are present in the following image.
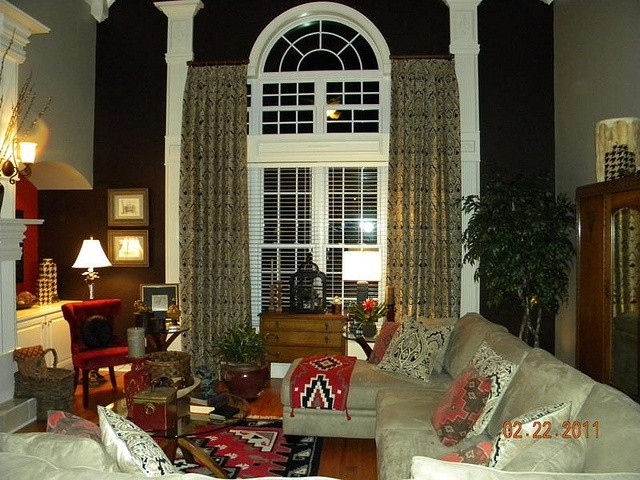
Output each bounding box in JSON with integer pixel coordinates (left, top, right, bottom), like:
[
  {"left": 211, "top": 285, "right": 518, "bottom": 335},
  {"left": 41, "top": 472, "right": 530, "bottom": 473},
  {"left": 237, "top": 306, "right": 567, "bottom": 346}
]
[{"left": 106, "top": 386, "right": 251, "bottom": 479}]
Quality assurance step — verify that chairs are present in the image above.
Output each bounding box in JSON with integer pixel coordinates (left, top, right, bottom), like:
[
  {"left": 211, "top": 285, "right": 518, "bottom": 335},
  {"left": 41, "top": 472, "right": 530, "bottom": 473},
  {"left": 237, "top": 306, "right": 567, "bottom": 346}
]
[{"left": 61, "top": 298, "right": 130, "bottom": 408}]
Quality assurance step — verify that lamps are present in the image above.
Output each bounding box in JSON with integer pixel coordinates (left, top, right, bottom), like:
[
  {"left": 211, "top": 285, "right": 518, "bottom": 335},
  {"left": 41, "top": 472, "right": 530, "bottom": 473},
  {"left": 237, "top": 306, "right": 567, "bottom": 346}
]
[
  {"left": 341, "top": 251, "right": 382, "bottom": 303},
  {"left": 2, "top": 137, "right": 37, "bottom": 184},
  {"left": 72, "top": 234, "right": 111, "bottom": 299}
]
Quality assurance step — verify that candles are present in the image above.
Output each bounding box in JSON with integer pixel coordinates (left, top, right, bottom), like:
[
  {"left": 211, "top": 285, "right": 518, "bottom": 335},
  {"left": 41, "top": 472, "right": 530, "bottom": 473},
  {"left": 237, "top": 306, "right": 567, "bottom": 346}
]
[
  {"left": 126, "top": 326, "right": 145, "bottom": 358},
  {"left": 276, "top": 254, "right": 281, "bottom": 281},
  {"left": 271, "top": 260, "right": 274, "bottom": 285}
]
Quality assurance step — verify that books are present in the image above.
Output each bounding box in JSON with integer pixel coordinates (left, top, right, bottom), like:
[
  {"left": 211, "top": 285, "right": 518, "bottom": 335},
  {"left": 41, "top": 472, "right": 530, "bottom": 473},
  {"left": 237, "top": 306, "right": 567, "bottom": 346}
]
[
  {"left": 189, "top": 400, "right": 226, "bottom": 414},
  {"left": 209, "top": 405, "right": 240, "bottom": 421},
  {"left": 190, "top": 413, "right": 210, "bottom": 421},
  {"left": 190, "top": 390, "right": 225, "bottom": 406}
]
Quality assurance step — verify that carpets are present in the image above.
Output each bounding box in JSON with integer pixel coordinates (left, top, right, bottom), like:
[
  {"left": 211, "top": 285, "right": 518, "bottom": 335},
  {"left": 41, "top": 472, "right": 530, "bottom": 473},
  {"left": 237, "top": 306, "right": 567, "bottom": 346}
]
[{"left": 126, "top": 414, "right": 322, "bottom": 476}]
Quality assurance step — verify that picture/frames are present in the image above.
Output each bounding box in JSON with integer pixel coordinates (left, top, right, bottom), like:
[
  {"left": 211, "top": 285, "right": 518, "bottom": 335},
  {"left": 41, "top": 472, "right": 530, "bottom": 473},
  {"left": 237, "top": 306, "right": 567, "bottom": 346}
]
[
  {"left": 139, "top": 283, "right": 178, "bottom": 318},
  {"left": 108, "top": 229, "right": 149, "bottom": 268},
  {"left": 106, "top": 187, "right": 149, "bottom": 227}
]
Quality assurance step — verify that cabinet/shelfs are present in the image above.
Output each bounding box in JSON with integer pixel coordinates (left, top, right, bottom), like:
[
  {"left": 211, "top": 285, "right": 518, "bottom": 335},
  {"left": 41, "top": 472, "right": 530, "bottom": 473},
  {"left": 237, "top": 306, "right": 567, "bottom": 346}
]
[
  {"left": 258, "top": 309, "right": 348, "bottom": 362},
  {"left": 16, "top": 300, "right": 81, "bottom": 373},
  {"left": 575, "top": 176, "right": 640, "bottom": 402}
]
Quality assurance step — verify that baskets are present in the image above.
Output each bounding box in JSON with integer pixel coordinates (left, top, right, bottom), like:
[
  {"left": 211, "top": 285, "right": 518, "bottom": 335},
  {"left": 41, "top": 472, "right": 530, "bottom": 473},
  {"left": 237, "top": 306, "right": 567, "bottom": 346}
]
[
  {"left": 147, "top": 350, "right": 192, "bottom": 379},
  {"left": 13, "top": 349, "right": 76, "bottom": 422}
]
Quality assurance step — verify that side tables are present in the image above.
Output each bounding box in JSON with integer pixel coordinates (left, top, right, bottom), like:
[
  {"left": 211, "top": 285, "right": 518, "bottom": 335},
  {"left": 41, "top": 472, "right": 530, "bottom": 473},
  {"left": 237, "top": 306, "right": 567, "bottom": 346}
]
[{"left": 135, "top": 324, "right": 190, "bottom": 353}]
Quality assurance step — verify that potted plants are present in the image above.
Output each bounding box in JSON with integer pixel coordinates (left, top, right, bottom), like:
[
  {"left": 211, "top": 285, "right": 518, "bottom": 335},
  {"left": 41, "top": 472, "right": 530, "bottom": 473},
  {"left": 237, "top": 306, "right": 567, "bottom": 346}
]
[{"left": 206, "top": 313, "right": 279, "bottom": 398}]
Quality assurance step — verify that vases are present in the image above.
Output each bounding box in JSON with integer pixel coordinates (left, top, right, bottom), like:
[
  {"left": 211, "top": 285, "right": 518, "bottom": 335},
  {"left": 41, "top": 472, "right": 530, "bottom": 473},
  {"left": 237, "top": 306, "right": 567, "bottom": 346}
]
[{"left": 362, "top": 325, "right": 377, "bottom": 337}]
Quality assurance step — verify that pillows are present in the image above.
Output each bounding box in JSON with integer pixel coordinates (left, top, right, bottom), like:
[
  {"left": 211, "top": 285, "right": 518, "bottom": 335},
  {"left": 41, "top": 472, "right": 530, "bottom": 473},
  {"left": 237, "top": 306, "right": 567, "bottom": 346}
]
[
  {"left": 431, "top": 361, "right": 492, "bottom": 446},
  {"left": 466, "top": 340, "right": 520, "bottom": 438},
  {"left": 490, "top": 403, "right": 572, "bottom": 468},
  {"left": 46, "top": 410, "right": 102, "bottom": 442},
  {"left": 441, "top": 441, "right": 490, "bottom": 465},
  {"left": 0, "top": 432, "right": 121, "bottom": 471},
  {"left": 96, "top": 404, "right": 180, "bottom": 475},
  {"left": 503, "top": 425, "right": 587, "bottom": 472},
  {"left": 377, "top": 314, "right": 454, "bottom": 381},
  {"left": 366, "top": 320, "right": 401, "bottom": 364}
]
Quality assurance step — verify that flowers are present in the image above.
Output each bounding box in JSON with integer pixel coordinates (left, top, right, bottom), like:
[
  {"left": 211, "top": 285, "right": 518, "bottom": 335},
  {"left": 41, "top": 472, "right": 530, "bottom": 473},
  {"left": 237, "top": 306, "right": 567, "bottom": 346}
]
[{"left": 345, "top": 299, "right": 387, "bottom": 326}]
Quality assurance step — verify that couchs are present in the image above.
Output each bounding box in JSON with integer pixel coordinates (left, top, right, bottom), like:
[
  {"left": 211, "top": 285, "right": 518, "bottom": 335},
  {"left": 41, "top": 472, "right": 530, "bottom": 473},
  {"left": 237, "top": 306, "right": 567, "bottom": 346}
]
[{"left": 280, "top": 312, "right": 639, "bottom": 480}]
